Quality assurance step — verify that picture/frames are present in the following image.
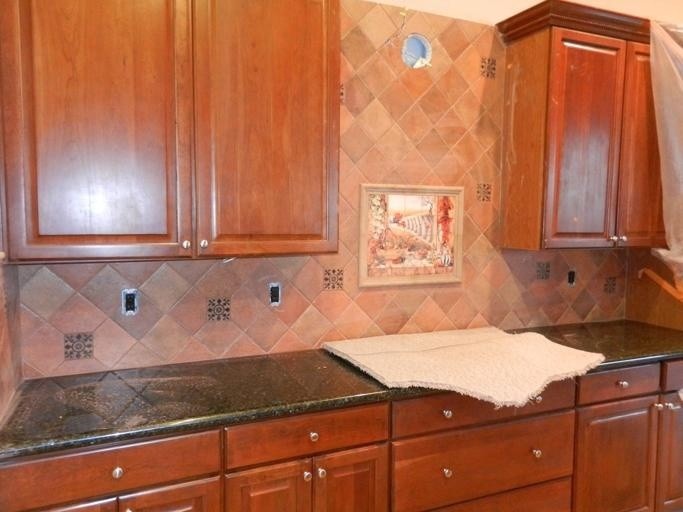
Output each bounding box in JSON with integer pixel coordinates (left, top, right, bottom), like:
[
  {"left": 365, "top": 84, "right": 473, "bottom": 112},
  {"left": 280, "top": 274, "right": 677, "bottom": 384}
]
[{"left": 358, "top": 183, "right": 465, "bottom": 290}]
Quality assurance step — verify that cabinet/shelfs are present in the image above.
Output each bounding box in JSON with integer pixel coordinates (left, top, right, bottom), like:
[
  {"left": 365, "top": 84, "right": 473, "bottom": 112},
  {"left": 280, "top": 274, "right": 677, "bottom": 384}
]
[
  {"left": 494, "top": 0, "right": 683, "bottom": 253},
  {"left": 573, "top": 358, "right": 682, "bottom": 511},
  {"left": 1, "top": 1, "right": 342, "bottom": 267},
  {"left": 223, "top": 402, "right": 390, "bottom": 512},
  {"left": 0, "top": 429, "right": 222, "bottom": 512},
  {"left": 391, "top": 375, "right": 576, "bottom": 512}
]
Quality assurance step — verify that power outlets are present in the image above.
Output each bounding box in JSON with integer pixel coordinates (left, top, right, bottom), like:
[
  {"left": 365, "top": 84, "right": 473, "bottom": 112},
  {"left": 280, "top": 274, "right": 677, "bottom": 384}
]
[
  {"left": 268, "top": 281, "right": 281, "bottom": 307},
  {"left": 122, "top": 287, "right": 139, "bottom": 317}
]
